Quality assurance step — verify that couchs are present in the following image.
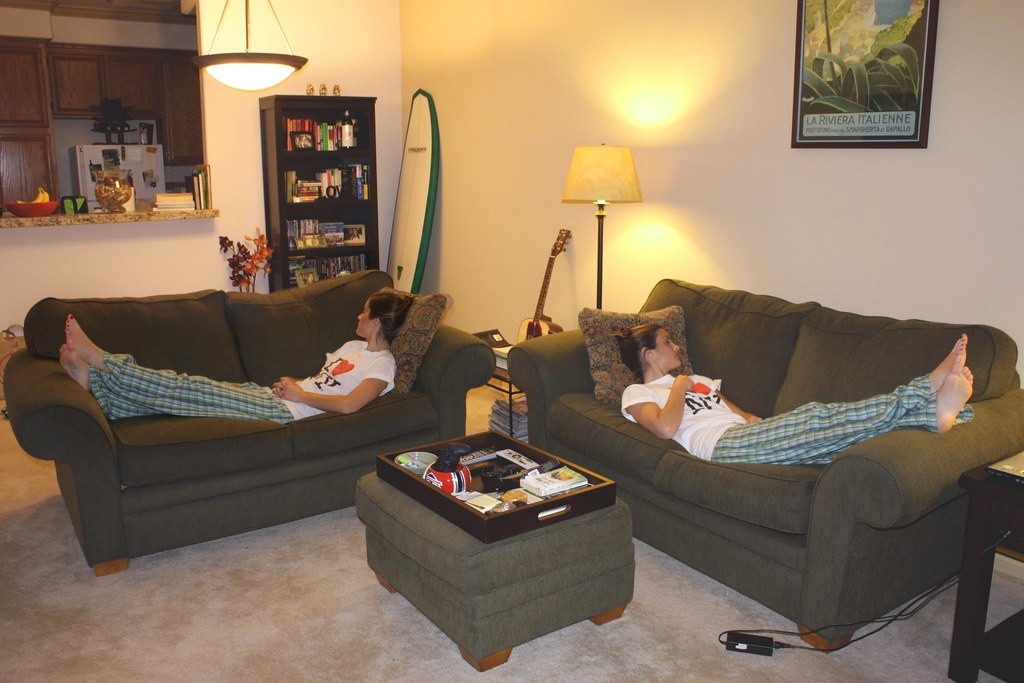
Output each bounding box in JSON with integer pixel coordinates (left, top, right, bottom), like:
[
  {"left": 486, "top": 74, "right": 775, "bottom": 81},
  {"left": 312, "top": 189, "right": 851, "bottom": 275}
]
[
  {"left": 4, "top": 268, "right": 496, "bottom": 576},
  {"left": 508, "top": 278, "right": 1023, "bottom": 651}
]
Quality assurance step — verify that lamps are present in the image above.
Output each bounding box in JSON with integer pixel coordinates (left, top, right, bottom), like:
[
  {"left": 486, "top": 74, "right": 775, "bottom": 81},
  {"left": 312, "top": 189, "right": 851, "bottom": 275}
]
[
  {"left": 560, "top": 146, "right": 642, "bottom": 311},
  {"left": 191, "top": 1, "right": 309, "bottom": 90}
]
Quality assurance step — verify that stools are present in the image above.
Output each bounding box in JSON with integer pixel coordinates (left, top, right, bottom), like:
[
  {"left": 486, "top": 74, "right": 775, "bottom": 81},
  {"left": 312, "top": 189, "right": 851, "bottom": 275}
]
[{"left": 356, "top": 472, "right": 636, "bottom": 671}]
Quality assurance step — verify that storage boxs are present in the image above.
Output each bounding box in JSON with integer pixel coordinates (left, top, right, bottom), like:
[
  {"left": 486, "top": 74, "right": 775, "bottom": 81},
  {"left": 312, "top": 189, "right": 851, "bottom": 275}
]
[{"left": 377, "top": 430, "right": 616, "bottom": 543}]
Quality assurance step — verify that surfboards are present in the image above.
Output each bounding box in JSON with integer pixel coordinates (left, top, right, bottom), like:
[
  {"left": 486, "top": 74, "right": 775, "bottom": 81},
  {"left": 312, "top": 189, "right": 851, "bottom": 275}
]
[{"left": 386, "top": 88, "right": 439, "bottom": 294}]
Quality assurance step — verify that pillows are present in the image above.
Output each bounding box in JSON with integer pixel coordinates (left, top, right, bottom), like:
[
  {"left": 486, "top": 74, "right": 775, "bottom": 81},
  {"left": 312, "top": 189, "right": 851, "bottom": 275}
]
[
  {"left": 374, "top": 289, "right": 447, "bottom": 395},
  {"left": 578, "top": 306, "right": 693, "bottom": 407}
]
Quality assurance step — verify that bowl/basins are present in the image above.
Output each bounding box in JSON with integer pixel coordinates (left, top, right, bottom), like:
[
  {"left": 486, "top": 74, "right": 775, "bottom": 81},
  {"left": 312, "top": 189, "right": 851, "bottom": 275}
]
[
  {"left": 425, "top": 464, "right": 471, "bottom": 496},
  {"left": 5, "top": 200, "right": 59, "bottom": 217},
  {"left": 395, "top": 451, "right": 438, "bottom": 474}
]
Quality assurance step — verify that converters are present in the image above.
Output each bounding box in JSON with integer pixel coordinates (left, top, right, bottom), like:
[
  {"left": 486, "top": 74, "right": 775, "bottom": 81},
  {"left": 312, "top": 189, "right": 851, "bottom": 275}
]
[{"left": 726, "top": 632, "right": 773, "bottom": 657}]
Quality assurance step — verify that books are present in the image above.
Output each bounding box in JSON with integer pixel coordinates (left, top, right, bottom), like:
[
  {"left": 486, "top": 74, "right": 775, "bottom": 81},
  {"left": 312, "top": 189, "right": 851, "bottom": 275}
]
[
  {"left": 519, "top": 466, "right": 588, "bottom": 497},
  {"left": 289, "top": 255, "right": 364, "bottom": 288},
  {"left": 151, "top": 165, "right": 211, "bottom": 211},
  {"left": 472, "top": 328, "right": 522, "bottom": 393},
  {"left": 286, "top": 119, "right": 342, "bottom": 151},
  {"left": 489, "top": 392, "right": 528, "bottom": 443},
  {"left": 288, "top": 219, "right": 343, "bottom": 248},
  {"left": 285, "top": 164, "right": 368, "bottom": 203}
]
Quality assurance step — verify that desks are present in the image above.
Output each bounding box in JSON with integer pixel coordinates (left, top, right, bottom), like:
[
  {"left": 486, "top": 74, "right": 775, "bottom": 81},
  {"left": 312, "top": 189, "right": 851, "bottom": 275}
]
[{"left": 949, "top": 448, "right": 1024, "bottom": 683}]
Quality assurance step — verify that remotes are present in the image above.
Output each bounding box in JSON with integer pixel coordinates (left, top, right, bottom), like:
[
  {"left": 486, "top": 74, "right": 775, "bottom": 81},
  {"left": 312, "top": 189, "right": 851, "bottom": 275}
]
[
  {"left": 496, "top": 449, "right": 540, "bottom": 472},
  {"left": 480, "top": 464, "right": 522, "bottom": 483},
  {"left": 460, "top": 447, "right": 498, "bottom": 466}
]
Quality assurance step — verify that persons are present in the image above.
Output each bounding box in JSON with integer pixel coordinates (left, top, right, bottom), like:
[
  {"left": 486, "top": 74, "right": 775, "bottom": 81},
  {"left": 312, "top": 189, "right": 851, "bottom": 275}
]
[
  {"left": 347, "top": 229, "right": 362, "bottom": 240},
  {"left": 609, "top": 324, "right": 976, "bottom": 465},
  {"left": 59, "top": 294, "right": 410, "bottom": 423}
]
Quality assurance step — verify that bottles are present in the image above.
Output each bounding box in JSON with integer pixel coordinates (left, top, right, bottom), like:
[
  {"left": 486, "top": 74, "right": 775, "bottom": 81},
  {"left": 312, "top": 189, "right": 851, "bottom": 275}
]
[{"left": 341, "top": 110, "right": 354, "bottom": 150}]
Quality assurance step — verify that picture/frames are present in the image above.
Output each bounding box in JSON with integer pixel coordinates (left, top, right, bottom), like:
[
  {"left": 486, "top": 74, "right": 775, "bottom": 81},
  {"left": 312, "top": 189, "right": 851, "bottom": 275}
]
[{"left": 791, "top": 0, "right": 941, "bottom": 149}]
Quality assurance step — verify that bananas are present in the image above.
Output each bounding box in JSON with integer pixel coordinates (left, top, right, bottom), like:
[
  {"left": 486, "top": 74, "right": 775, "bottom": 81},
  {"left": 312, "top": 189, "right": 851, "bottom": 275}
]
[{"left": 16, "top": 187, "right": 49, "bottom": 203}]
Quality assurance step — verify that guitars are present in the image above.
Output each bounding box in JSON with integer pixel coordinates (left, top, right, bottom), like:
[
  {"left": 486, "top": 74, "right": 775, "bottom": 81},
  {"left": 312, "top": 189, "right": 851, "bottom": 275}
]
[{"left": 517, "top": 228, "right": 573, "bottom": 344}]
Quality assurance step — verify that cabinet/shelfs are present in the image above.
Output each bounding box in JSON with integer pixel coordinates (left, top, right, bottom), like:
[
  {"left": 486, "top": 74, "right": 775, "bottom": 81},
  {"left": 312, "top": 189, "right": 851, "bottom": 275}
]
[
  {"left": 259, "top": 94, "right": 378, "bottom": 296},
  {"left": 0, "top": 0, "right": 224, "bottom": 229}
]
